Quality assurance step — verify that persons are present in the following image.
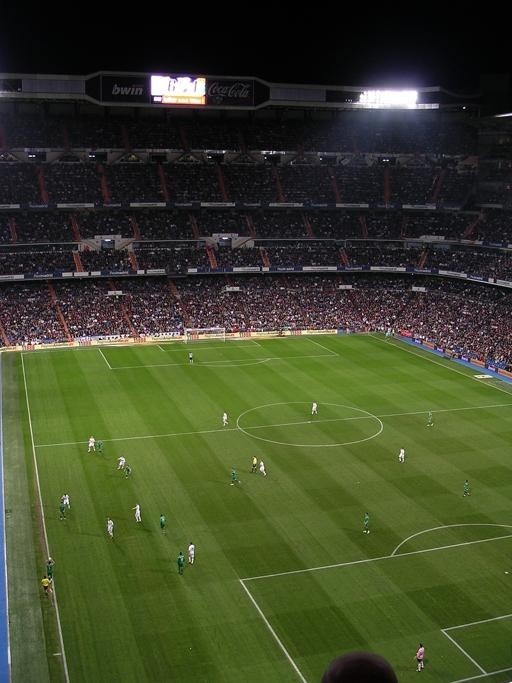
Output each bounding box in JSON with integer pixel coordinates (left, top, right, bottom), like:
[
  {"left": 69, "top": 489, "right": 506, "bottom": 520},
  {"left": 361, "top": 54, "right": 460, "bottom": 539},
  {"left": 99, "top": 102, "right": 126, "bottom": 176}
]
[
  {"left": 427, "top": 409, "right": 434, "bottom": 427},
  {"left": 463, "top": 479, "right": 471, "bottom": 497},
  {"left": 311, "top": 401, "right": 318, "bottom": 415},
  {"left": 41, "top": 558, "right": 56, "bottom": 607},
  {"left": 251, "top": 456, "right": 258, "bottom": 474},
  {"left": 59, "top": 492, "right": 71, "bottom": 520},
  {"left": 230, "top": 467, "right": 240, "bottom": 487},
  {"left": 259, "top": 458, "right": 266, "bottom": 476},
  {"left": 362, "top": 512, "right": 370, "bottom": 534},
  {"left": 107, "top": 504, "right": 195, "bottom": 575},
  {"left": 320, "top": 650, "right": 398, "bottom": 683},
  {"left": 86, "top": 435, "right": 132, "bottom": 479},
  {"left": 398, "top": 446, "right": 404, "bottom": 464},
  {"left": 222, "top": 412, "right": 229, "bottom": 426},
  {"left": 414, "top": 643, "right": 425, "bottom": 673}
]
